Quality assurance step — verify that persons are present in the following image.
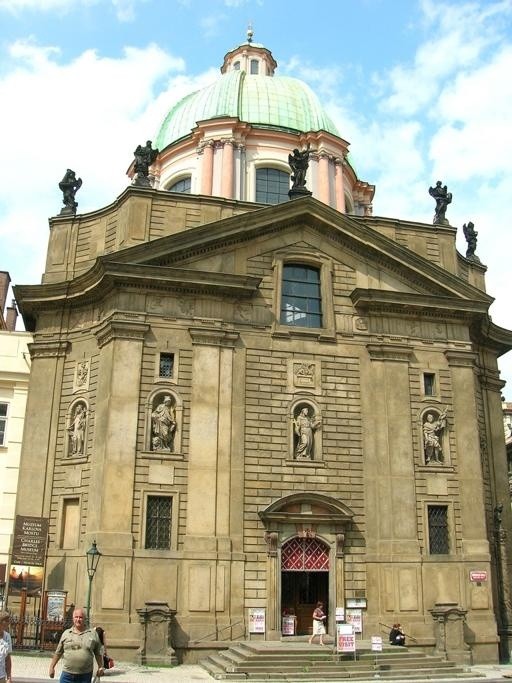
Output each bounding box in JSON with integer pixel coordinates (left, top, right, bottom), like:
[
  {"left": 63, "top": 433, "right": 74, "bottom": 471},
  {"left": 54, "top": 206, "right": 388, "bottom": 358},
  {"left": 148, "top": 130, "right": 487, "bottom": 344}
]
[
  {"left": 134, "top": 140, "right": 159, "bottom": 177},
  {"left": 433, "top": 180, "right": 449, "bottom": 219},
  {"left": 66, "top": 405, "right": 86, "bottom": 455},
  {"left": 308, "top": 601, "right": 327, "bottom": 646},
  {"left": 422, "top": 413, "right": 443, "bottom": 464},
  {"left": 466, "top": 221, "right": 478, "bottom": 255},
  {"left": 48, "top": 608, "right": 105, "bottom": 683},
  {"left": 93, "top": 626, "right": 108, "bottom": 663},
  {"left": 288, "top": 147, "right": 309, "bottom": 188},
  {"left": 389, "top": 623, "right": 406, "bottom": 646},
  {"left": 59, "top": 169, "right": 83, "bottom": 207},
  {"left": 294, "top": 406, "right": 322, "bottom": 459},
  {"left": 0, "top": 612, "right": 13, "bottom": 683},
  {"left": 283, "top": 605, "right": 298, "bottom": 636},
  {"left": 150, "top": 395, "right": 177, "bottom": 447}
]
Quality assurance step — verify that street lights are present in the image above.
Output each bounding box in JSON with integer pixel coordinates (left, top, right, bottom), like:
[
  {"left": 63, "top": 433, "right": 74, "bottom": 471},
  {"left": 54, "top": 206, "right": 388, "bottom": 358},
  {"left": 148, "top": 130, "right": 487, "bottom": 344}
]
[{"left": 85, "top": 538, "right": 101, "bottom": 626}]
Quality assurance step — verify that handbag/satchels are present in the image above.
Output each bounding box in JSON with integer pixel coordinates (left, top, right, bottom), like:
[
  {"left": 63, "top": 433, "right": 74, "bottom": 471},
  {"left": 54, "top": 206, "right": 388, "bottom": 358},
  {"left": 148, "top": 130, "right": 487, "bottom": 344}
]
[{"left": 104, "top": 656, "right": 114, "bottom": 669}]
